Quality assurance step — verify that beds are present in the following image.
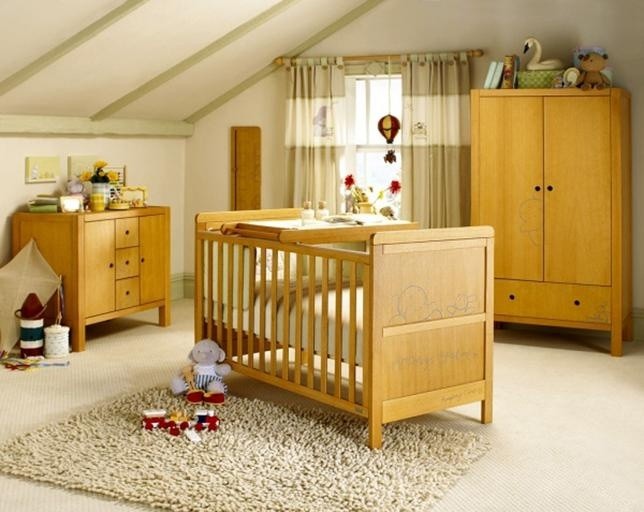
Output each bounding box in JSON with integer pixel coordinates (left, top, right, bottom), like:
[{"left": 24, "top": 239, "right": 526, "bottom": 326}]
[{"left": 193, "top": 207, "right": 494, "bottom": 449}]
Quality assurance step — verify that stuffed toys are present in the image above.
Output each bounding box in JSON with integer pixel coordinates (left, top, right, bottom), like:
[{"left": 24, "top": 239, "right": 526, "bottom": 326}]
[
  {"left": 170, "top": 339, "right": 231, "bottom": 404},
  {"left": 142, "top": 408, "right": 221, "bottom": 436},
  {"left": 576, "top": 52, "right": 610, "bottom": 91}
]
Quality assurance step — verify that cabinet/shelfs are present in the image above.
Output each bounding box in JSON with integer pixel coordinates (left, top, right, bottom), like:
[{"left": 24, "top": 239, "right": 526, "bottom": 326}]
[
  {"left": 469, "top": 88, "right": 630, "bottom": 357},
  {"left": 12, "top": 208, "right": 171, "bottom": 352}
]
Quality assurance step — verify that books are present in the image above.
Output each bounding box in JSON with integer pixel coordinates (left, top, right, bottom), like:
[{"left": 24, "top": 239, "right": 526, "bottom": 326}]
[{"left": 483, "top": 54, "right": 517, "bottom": 89}]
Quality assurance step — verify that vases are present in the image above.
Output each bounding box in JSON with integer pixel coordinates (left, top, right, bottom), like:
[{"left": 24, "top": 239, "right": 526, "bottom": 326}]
[
  {"left": 89, "top": 183, "right": 116, "bottom": 210},
  {"left": 354, "top": 203, "right": 373, "bottom": 214}
]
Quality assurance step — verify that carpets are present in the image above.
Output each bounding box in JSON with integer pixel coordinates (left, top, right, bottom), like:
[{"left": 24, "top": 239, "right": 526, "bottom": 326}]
[{"left": 0, "top": 385, "right": 487, "bottom": 511}]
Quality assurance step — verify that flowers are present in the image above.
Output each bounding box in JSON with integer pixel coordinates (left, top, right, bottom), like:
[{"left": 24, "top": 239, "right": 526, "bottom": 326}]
[
  {"left": 344, "top": 175, "right": 403, "bottom": 202},
  {"left": 78, "top": 161, "right": 118, "bottom": 183}
]
